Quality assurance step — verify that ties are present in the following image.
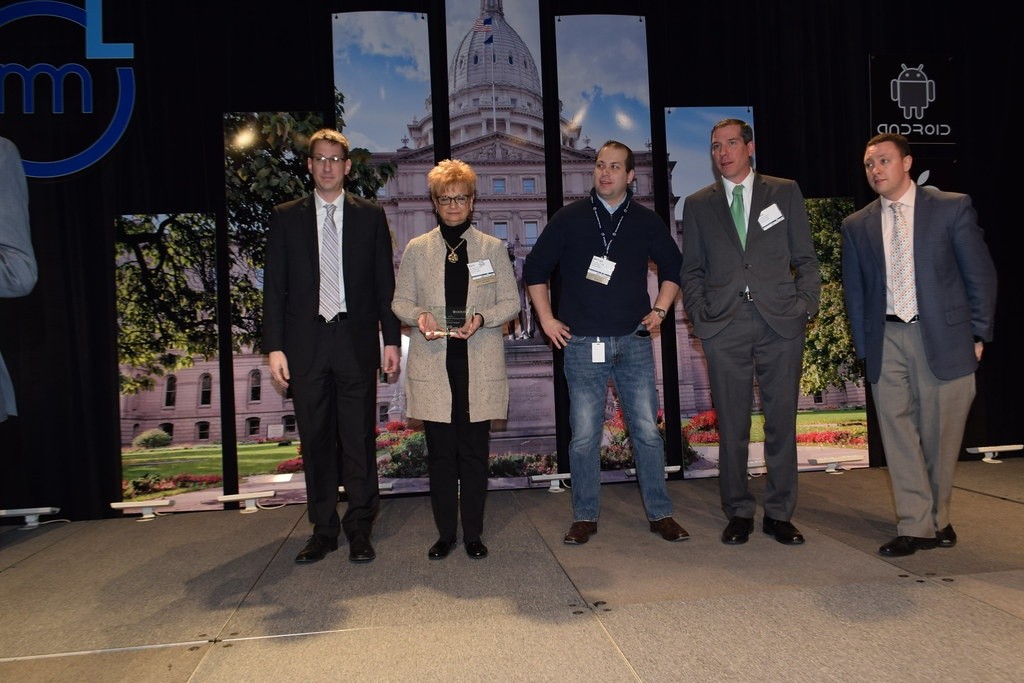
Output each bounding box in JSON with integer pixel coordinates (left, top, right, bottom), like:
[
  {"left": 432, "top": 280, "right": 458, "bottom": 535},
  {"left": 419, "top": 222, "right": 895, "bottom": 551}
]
[
  {"left": 730, "top": 186, "right": 746, "bottom": 250},
  {"left": 890, "top": 202, "right": 917, "bottom": 323},
  {"left": 319, "top": 204, "right": 341, "bottom": 321}
]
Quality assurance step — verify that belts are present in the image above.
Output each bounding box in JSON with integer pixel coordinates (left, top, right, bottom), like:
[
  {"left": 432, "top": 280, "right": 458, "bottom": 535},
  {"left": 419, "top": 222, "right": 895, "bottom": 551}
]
[
  {"left": 886, "top": 314, "right": 920, "bottom": 322},
  {"left": 743, "top": 291, "right": 755, "bottom": 304}
]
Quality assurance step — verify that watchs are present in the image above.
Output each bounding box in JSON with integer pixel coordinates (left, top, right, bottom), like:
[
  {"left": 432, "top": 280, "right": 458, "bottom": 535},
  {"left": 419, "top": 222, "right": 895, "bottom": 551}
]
[{"left": 653, "top": 307, "right": 665, "bottom": 320}]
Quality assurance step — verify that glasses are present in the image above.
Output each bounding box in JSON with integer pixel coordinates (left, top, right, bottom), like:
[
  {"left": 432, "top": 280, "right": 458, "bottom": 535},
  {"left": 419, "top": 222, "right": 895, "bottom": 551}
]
[
  {"left": 436, "top": 195, "right": 470, "bottom": 206},
  {"left": 314, "top": 154, "right": 346, "bottom": 164}
]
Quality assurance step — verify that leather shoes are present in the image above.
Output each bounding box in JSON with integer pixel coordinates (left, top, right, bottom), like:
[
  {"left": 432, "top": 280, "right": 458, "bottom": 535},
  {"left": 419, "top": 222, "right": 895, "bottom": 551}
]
[
  {"left": 565, "top": 521, "right": 598, "bottom": 544},
  {"left": 463, "top": 535, "right": 488, "bottom": 559},
  {"left": 295, "top": 533, "right": 338, "bottom": 561},
  {"left": 762, "top": 517, "right": 805, "bottom": 545},
  {"left": 347, "top": 529, "right": 375, "bottom": 560},
  {"left": 935, "top": 523, "right": 957, "bottom": 547},
  {"left": 428, "top": 535, "right": 457, "bottom": 559},
  {"left": 722, "top": 517, "right": 754, "bottom": 544},
  {"left": 650, "top": 516, "right": 690, "bottom": 542},
  {"left": 879, "top": 535, "right": 937, "bottom": 556}
]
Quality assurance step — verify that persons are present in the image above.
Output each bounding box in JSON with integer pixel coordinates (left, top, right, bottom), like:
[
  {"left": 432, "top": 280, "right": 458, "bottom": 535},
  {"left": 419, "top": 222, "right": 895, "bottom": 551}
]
[
  {"left": 392, "top": 159, "right": 521, "bottom": 560},
  {"left": 679, "top": 119, "right": 821, "bottom": 545},
  {"left": 259, "top": 129, "right": 401, "bottom": 563},
  {"left": 0, "top": 138, "right": 38, "bottom": 424},
  {"left": 842, "top": 133, "right": 997, "bottom": 557},
  {"left": 523, "top": 141, "right": 690, "bottom": 544}
]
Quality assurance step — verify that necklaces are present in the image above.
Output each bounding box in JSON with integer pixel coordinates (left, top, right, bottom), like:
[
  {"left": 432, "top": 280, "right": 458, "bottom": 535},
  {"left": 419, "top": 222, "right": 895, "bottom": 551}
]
[{"left": 441, "top": 238, "right": 465, "bottom": 264}]
